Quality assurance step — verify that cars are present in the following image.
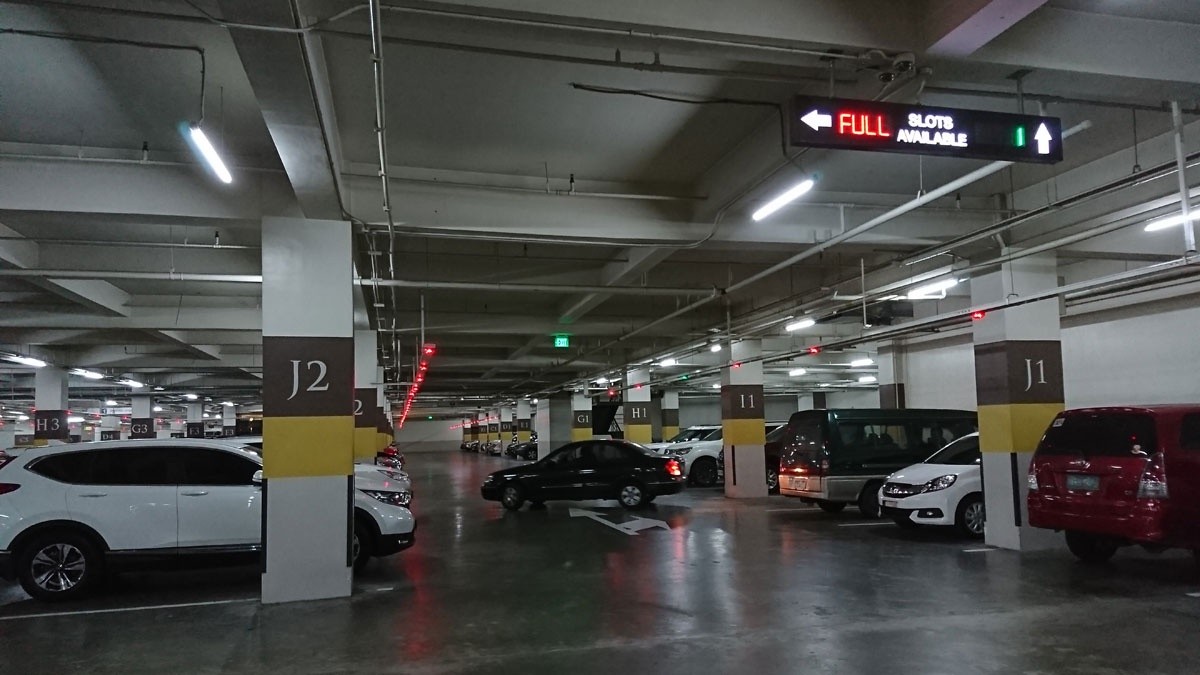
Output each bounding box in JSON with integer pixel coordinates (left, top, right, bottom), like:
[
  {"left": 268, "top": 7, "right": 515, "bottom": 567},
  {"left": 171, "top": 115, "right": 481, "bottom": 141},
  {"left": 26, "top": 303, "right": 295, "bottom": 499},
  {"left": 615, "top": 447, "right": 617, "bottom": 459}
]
[
  {"left": 643, "top": 419, "right": 789, "bottom": 495},
  {"left": 193, "top": 435, "right": 417, "bottom": 510},
  {"left": 460, "top": 436, "right": 542, "bottom": 464},
  {"left": 878, "top": 431, "right": 984, "bottom": 541},
  {"left": 479, "top": 439, "right": 688, "bottom": 512}
]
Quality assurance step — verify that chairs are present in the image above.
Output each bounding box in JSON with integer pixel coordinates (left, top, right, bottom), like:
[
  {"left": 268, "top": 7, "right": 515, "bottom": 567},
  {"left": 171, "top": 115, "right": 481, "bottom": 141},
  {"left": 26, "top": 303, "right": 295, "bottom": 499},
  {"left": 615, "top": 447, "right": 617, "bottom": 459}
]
[{"left": 850, "top": 433, "right": 900, "bottom": 451}]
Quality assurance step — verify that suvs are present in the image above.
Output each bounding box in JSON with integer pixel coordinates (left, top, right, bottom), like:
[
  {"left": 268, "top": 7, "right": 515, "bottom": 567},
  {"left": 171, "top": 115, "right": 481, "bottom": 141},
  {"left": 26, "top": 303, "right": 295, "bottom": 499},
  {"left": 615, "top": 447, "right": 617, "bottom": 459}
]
[
  {"left": 1025, "top": 403, "right": 1200, "bottom": 566},
  {"left": 0, "top": 438, "right": 420, "bottom": 606},
  {"left": 778, "top": 407, "right": 980, "bottom": 518}
]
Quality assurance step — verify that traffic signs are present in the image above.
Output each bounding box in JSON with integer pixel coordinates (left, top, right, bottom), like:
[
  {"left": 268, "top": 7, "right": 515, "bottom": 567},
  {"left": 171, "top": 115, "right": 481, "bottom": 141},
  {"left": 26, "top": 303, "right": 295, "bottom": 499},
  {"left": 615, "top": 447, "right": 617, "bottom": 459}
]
[{"left": 789, "top": 92, "right": 1065, "bottom": 166}]
